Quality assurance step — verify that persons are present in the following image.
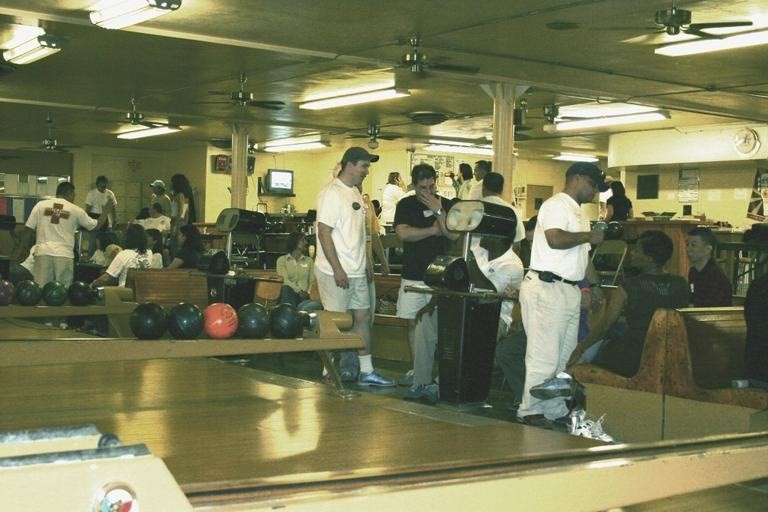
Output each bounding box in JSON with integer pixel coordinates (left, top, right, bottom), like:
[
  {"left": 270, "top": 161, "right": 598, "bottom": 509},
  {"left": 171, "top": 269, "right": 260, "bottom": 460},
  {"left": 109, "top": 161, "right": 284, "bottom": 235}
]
[
  {"left": 743, "top": 274, "right": 766, "bottom": 390},
  {"left": 686, "top": 230, "right": 735, "bottom": 307},
  {"left": 16, "top": 173, "right": 200, "bottom": 337},
  {"left": 519, "top": 162, "right": 688, "bottom": 431},
  {"left": 276, "top": 147, "right": 396, "bottom": 386},
  {"left": 381, "top": 160, "right": 538, "bottom": 408}
]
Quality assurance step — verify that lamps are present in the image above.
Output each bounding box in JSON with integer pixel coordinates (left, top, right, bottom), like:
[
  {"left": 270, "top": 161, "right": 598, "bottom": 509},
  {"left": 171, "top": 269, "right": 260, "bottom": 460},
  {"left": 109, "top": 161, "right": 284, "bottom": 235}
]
[
  {"left": 3, "top": 36, "right": 68, "bottom": 69},
  {"left": 426, "top": 26, "right": 767, "bottom": 158},
  {"left": 88, "top": 0, "right": 182, "bottom": 29}
]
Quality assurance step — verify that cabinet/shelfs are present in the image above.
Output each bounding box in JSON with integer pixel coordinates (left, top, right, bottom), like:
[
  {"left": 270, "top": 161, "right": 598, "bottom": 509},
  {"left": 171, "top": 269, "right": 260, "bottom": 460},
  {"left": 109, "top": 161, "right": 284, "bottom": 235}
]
[{"left": 106, "top": 180, "right": 142, "bottom": 223}]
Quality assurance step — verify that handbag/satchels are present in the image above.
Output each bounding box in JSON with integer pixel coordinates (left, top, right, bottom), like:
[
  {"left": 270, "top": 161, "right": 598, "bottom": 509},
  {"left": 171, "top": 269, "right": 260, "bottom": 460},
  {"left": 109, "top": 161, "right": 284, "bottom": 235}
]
[
  {"left": 376, "top": 286, "right": 400, "bottom": 314},
  {"left": 199, "top": 249, "right": 230, "bottom": 274}
]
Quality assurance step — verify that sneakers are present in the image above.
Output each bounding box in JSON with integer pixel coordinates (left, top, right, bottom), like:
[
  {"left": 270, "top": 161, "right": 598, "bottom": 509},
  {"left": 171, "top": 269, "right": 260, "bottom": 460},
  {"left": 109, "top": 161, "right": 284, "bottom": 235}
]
[{"left": 322, "top": 369, "right": 614, "bottom": 442}]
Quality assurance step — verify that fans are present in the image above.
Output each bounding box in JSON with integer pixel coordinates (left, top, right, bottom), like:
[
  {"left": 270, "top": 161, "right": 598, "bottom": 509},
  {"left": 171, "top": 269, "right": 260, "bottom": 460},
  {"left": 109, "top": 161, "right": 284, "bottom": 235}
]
[
  {"left": 352, "top": 39, "right": 478, "bottom": 85},
  {"left": 586, "top": 0, "right": 752, "bottom": 39},
  {"left": 94, "top": 99, "right": 184, "bottom": 133},
  {"left": 189, "top": 77, "right": 287, "bottom": 112},
  {"left": 21, "top": 123, "right": 80, "bottom": 153}
]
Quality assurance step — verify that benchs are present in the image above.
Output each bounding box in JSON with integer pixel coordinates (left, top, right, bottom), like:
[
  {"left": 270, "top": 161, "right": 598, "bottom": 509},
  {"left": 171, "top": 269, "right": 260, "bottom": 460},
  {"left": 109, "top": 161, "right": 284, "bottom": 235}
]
[{"left": 115, "top": 236, "right": 767, "bottom": 410}]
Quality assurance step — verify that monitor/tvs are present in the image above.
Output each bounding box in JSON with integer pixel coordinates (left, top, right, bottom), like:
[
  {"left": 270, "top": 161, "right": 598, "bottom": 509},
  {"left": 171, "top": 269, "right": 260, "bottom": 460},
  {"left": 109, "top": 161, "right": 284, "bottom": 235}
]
[{"left": 265, "top": 169, "right": 294, "bottom": 193}]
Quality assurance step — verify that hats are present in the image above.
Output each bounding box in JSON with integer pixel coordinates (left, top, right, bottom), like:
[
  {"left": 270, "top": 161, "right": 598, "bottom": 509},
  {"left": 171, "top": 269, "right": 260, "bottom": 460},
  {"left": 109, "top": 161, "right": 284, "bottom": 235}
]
[
  {"left": 566, "top": 162, "right": 605, "bottom": 185},
  {"left": 342, "top": 147, "right": 379, "bottom": 162},
  {"left": 150, "top": 180, "right": 165, "bottom": 188}
]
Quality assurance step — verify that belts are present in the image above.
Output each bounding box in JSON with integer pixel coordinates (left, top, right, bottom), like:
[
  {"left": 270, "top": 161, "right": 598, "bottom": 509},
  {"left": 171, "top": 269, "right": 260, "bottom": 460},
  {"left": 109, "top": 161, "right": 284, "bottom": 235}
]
[{"left": 554, "top": 275, "right": 577, "bottom": 286}]
